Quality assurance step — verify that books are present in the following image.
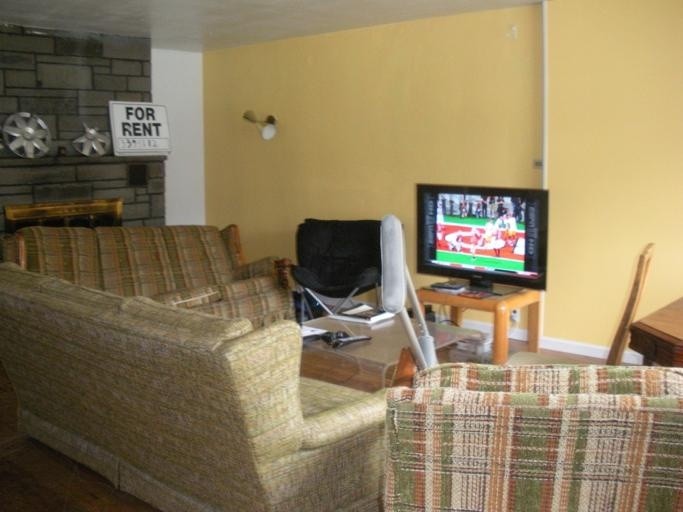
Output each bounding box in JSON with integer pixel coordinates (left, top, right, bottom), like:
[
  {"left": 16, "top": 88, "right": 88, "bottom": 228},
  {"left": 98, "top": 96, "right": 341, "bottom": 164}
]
[
  {"left": 328, "top": 302, "right": 395, "bottom": 324},
  {"left": 457, "top": 333, "right": 493, "bottom": 355}
]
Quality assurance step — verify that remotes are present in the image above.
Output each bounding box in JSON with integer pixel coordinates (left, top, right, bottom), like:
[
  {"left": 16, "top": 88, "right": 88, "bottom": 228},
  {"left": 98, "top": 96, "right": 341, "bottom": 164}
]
[{"left": 333, "top": 335, "right": 372, "bottom": 347}]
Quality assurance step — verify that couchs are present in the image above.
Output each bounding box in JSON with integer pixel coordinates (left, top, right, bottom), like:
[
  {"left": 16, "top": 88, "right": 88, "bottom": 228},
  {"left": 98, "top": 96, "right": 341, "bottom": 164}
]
[
  {"left": 2, "top": 224, "right": 296, "bottom": 328},
  {"left": 0, "top": 261, "right": 393, "bottom": 512}
]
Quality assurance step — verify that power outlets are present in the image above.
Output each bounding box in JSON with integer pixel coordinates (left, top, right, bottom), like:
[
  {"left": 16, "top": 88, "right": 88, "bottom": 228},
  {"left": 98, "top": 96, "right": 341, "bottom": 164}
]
[{"left": 511, "top": 310, "right": 520, "bottom": 323}]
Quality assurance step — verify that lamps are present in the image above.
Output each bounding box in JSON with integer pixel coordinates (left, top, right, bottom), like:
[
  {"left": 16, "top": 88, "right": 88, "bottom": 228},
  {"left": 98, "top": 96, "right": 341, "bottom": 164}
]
[{"left": 242, "top": 111, "right": 276, "bottom": 140}]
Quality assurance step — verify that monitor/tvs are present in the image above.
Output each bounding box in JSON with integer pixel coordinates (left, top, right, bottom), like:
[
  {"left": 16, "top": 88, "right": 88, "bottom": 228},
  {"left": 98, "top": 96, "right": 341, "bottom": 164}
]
[{"left": 416, "top": 183, "right": 548, "bottom": 290}]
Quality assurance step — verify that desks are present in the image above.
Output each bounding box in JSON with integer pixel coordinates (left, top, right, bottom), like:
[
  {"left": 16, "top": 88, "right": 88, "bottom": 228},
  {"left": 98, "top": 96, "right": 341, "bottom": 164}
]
[
  {"left": 629, "top": 298, "right": 683, "bottom": 367},
  {"left": 415, "top": 280, "right": 546, "bottom": 364}
]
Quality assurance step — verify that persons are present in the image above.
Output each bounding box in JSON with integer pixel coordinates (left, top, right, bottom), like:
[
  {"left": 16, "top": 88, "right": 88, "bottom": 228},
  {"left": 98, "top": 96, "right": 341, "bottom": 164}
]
[{"left": 436, "top": 194, "right": 526, "bottom": 257}]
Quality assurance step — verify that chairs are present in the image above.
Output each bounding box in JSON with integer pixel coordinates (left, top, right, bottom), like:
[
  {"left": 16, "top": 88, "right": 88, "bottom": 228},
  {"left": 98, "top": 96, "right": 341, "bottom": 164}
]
[
  {"left": 507, "top": 243, "right": 655, "bottom": 365},
  {"left": 4, "top": 199, "right": 123, "bottom": 233},
  {"left": 383, "top": 347, "right": 683, "bottom": 512}
]
